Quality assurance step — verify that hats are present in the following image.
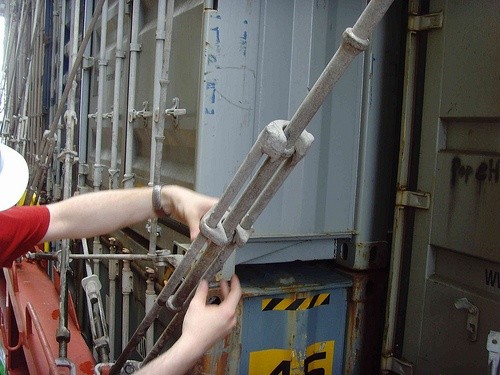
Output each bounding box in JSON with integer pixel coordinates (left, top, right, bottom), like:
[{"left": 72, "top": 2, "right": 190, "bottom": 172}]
[{"left": 0, "top": 142, "right": 29, "bottom": 211}]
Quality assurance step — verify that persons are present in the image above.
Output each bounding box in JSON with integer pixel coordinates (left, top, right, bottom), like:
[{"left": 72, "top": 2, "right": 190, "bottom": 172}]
[{"left": 1, "top": 142, "right": 242, "bottom": 375}]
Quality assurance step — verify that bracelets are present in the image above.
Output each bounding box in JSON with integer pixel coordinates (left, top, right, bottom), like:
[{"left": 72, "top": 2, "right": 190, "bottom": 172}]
[{"left": 152, "top": 183, "right": 172, "bottom": 218}]
